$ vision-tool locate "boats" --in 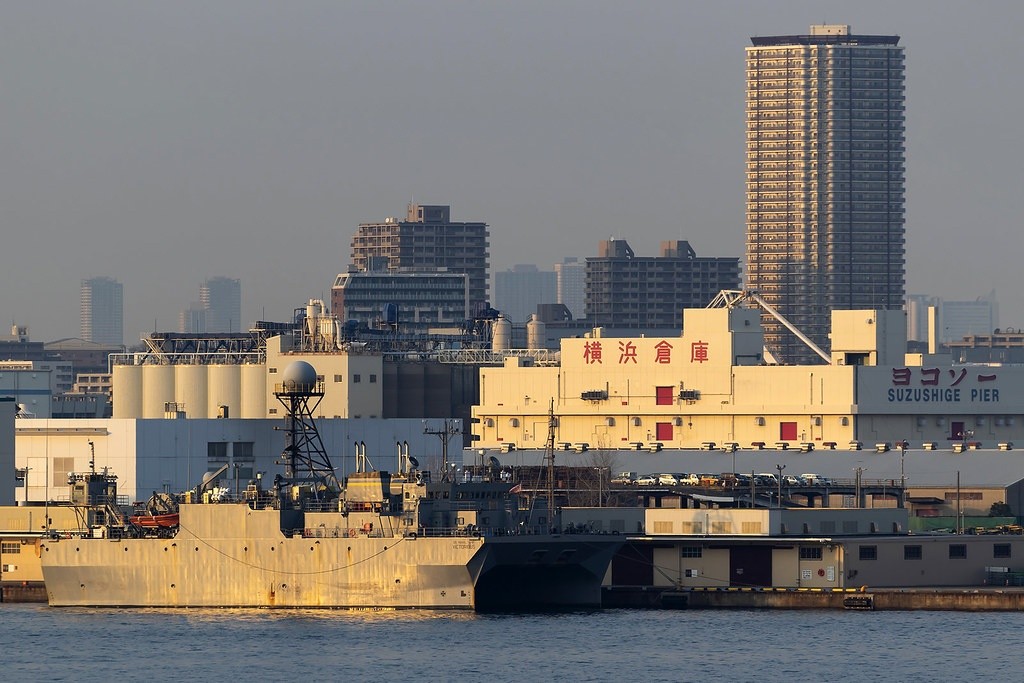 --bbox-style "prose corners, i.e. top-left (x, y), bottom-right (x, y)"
top-left (40, 396), bottom-right (627, 613)
